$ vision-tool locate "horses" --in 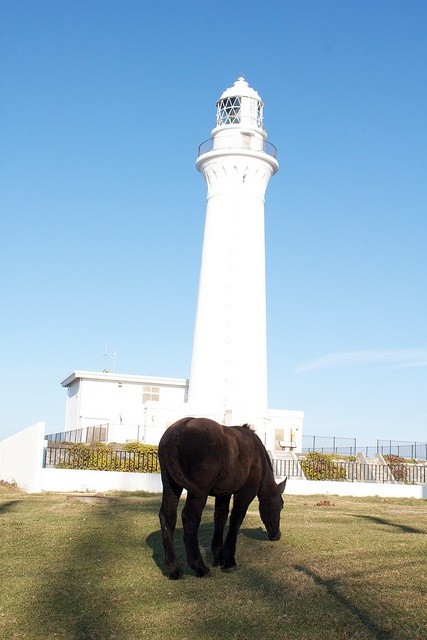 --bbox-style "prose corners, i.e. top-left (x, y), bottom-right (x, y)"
top-left (158, 417), bottom-right (288, 581)
